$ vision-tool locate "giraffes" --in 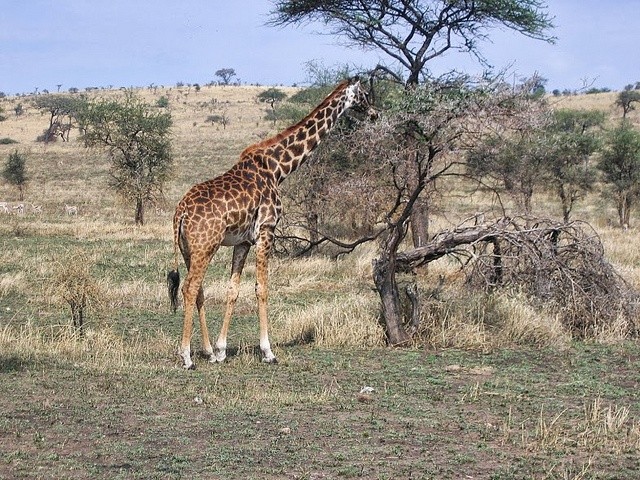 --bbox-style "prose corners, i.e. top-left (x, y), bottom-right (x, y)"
top-left (166, 73), bottom-right (380, 371)
